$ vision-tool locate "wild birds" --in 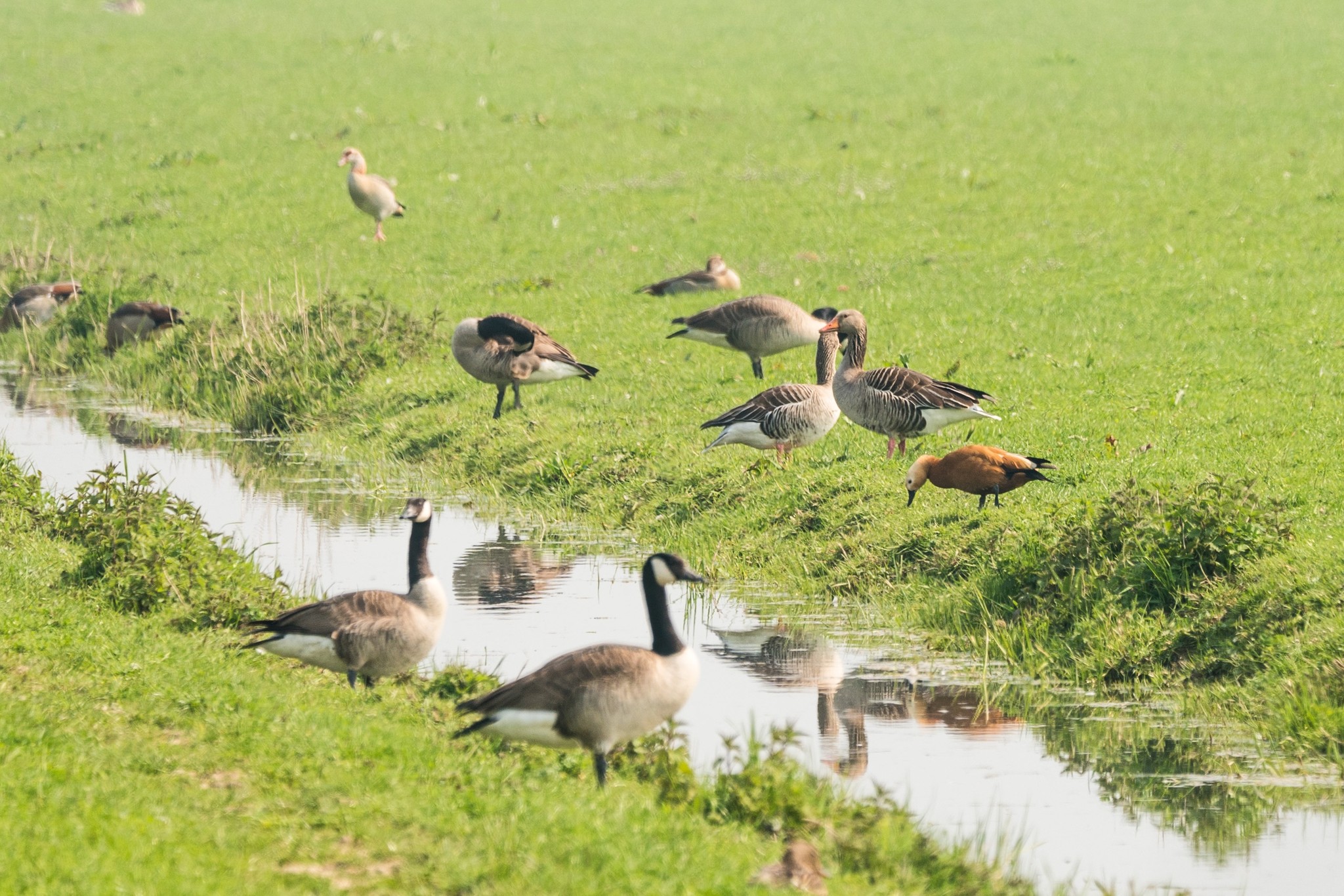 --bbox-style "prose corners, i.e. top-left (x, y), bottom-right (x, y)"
top-left (454, 518), bottom-right (574, 613)
top-left (818, 309), bottom-right (1002, 462)
top-left (821, 648), bottom-right (1027, 777)
top-left (451, 312), bottom-right (598, 419)
top-left (703, 604), bottom-right (841, 738)
top-left (237, 497), bottom-right (449, 690)
top-left (905, 445), bottom-right (1059, 514)
top-left (448, 553), bottom-right (705, 792)
top-left (699, 331), bottom-right (842, 465)
top-left (634, 254), bottom-right (741, 298)
top-left (665, 294), bottom-right (840, 379)
top-left (338, 148), bottom-right (406, 242)
